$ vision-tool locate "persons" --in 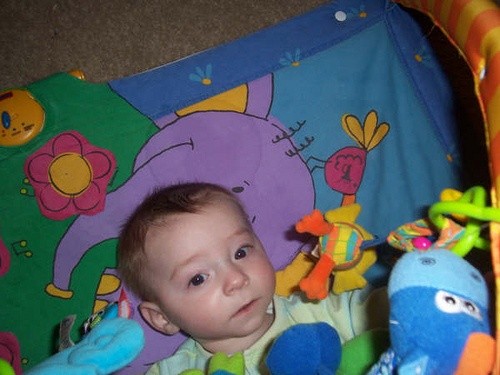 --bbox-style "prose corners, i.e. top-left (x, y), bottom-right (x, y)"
top-left (116, 176), bottom-right (388, 375)
